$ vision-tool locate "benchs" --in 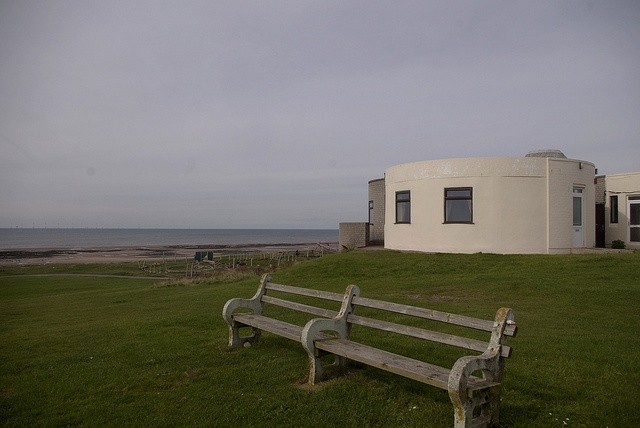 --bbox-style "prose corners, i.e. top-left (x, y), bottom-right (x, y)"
top-left (222, 273), bottom-right (518, 428)
